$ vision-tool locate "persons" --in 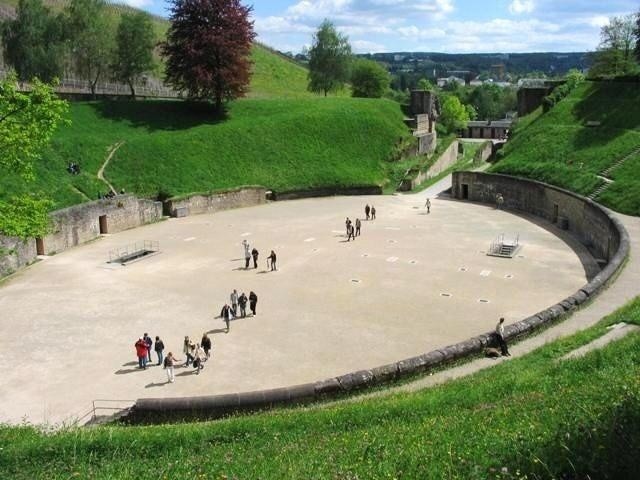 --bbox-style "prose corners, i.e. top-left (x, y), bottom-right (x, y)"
top-left (425, 198), bottom-right (431, 213)
top-left (496, 193), bottom-right (504, 210)
top-left (365, 204), bottom-right (370, 220)
top-left (135, 338), bottom-right (148, 370)
top-left (183, 333), bottom-right (211, 374)
top-left (252, 248), bottom-right (258, 268)
top-left (268, 250), bottom-right (276, 271)
top-left (496, 317), bottom-right (511, 356)
top-left (143, 333), bottom-right (152, 362)
top-left (371, 205), bottom-right (376, 220)
top-left (346, 217), bottom-right (361, 241)
top-left (243, 239), bottom-right (251, 267)
top-left (155, 336), bottom-right (164, 365)
top-left (163, 352), bottom-right (182, 383)
top-left (221, 289), bottom-right (257, 333)
